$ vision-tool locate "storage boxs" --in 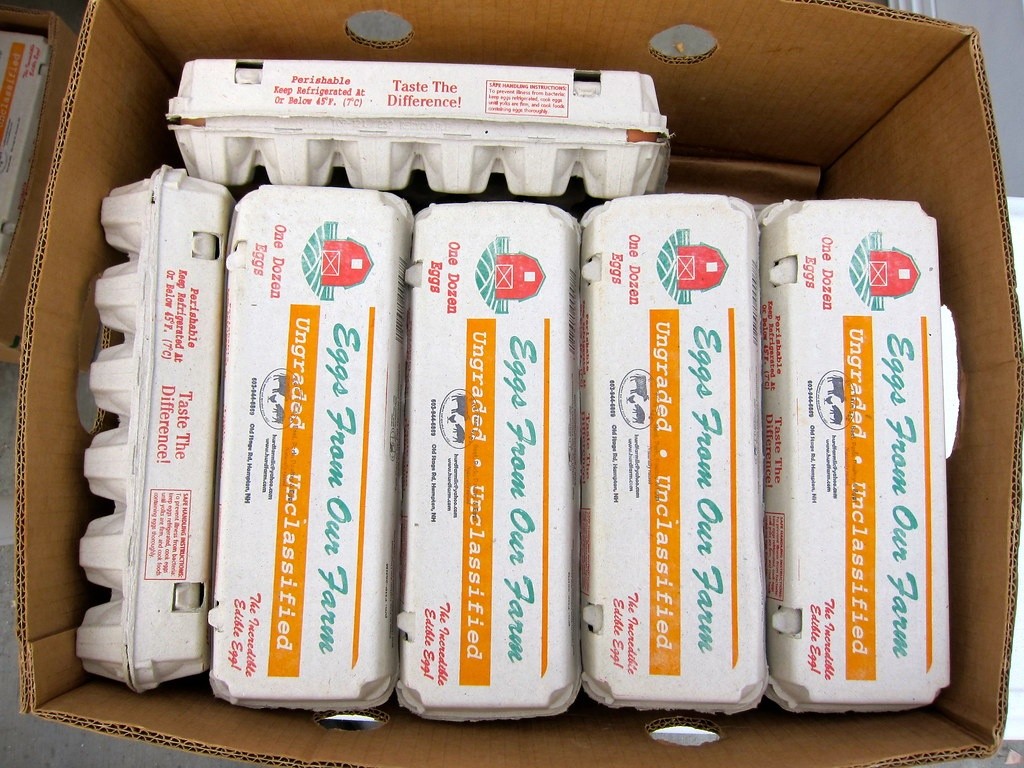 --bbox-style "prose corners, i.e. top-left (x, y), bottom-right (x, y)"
top-left (0, 0), bottom-right (1024, 768)
